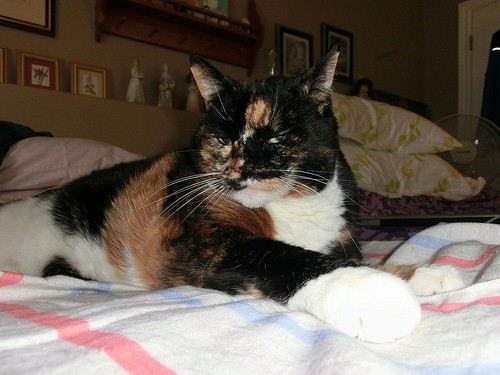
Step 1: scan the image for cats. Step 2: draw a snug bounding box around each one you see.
[0,44,465,336]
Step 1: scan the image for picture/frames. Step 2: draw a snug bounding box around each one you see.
[0,0,56,38]
[16,48,65,92]
[275,22,314,82]
[320,22,354,86]
[71,60,110,99]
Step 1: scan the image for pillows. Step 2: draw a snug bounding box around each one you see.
[331,92,487,202]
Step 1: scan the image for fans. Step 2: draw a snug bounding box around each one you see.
[437,113,500,187]
